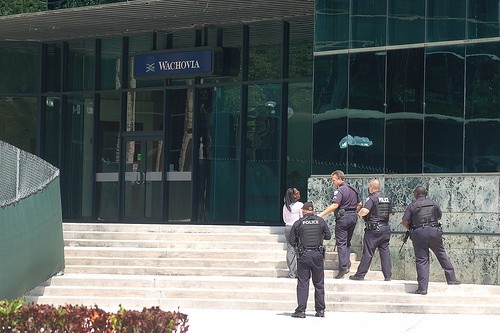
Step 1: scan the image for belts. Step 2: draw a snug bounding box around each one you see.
[306,247,317,251]
[345,208,356,211]
[418,224,438,227]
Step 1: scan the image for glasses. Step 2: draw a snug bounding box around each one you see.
[294,191,299,195]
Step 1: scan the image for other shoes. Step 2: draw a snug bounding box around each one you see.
[385,277,391,280]
[315,313,324,317]
[349,275,364,280]
[448,281,462,284]
[335,269,349,279]
[291,312,305,318]
[415,289,427,294]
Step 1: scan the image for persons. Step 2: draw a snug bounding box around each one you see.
[401,186,461,295]
[349,179,393,281]
[283,188,306,279]
[289,202,331,318]
[318,170,362,278]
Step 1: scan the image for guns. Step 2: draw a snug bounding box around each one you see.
[399,230,410,253]
[333,237,337,252]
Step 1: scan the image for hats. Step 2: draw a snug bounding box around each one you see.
[301,201,314,211]
